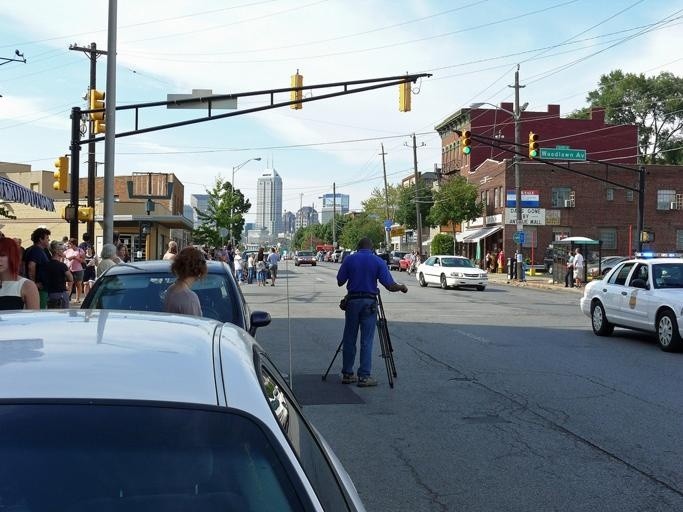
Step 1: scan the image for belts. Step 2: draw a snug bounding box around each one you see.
[347,290,379,300]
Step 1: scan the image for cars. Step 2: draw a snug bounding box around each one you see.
[324,250,333,262]
[399,254,428,272]
[588,257,622,276]
[416,255,488,291]
[295,251,316,266]
[80,261,271,337]
[331,250,342,262]
[0,310,363,512]
[580,252,683,352]
[601,257,635,275]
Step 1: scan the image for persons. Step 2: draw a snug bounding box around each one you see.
[0,238,40,310]
[45,240,73,309]
[564,251,575,288]
[165,246,208,317]
[82,245,98,297]
[407,249,421,275]
[116,243,131,263]
[163,241,177,260]
[97,244,117,278]
[78,233,91,252]
[336,237,408,387]
[63,236,69,246]
[187,241,192,246]
[573,248,584,288]
[253,248,268,288]
[234,251,245,283]
[66,238,85,303]
[22,228,52,309]
[200,243,235,272]
[267,247,280,286]
[247,253,254,284]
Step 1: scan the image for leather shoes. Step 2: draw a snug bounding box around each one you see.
[356,376,377,387]
[341,373,357,384]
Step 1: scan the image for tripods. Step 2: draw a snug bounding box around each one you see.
[321,288,397,389]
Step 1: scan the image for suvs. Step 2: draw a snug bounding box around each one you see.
[544,247,569,274]
[387,250,409,270]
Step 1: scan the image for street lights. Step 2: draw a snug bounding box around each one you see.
[469,102,523,282]
[318,197,336,250]
[230,157,261,246]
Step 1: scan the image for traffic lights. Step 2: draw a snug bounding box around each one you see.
[87,89,105,121]
[92,116,105,135]
[53,157,68,192]
[399,78,412,112]
[529,131,539,160]
[462,130,472,154]
[62,205,94,223]
[291,69,303,109]
